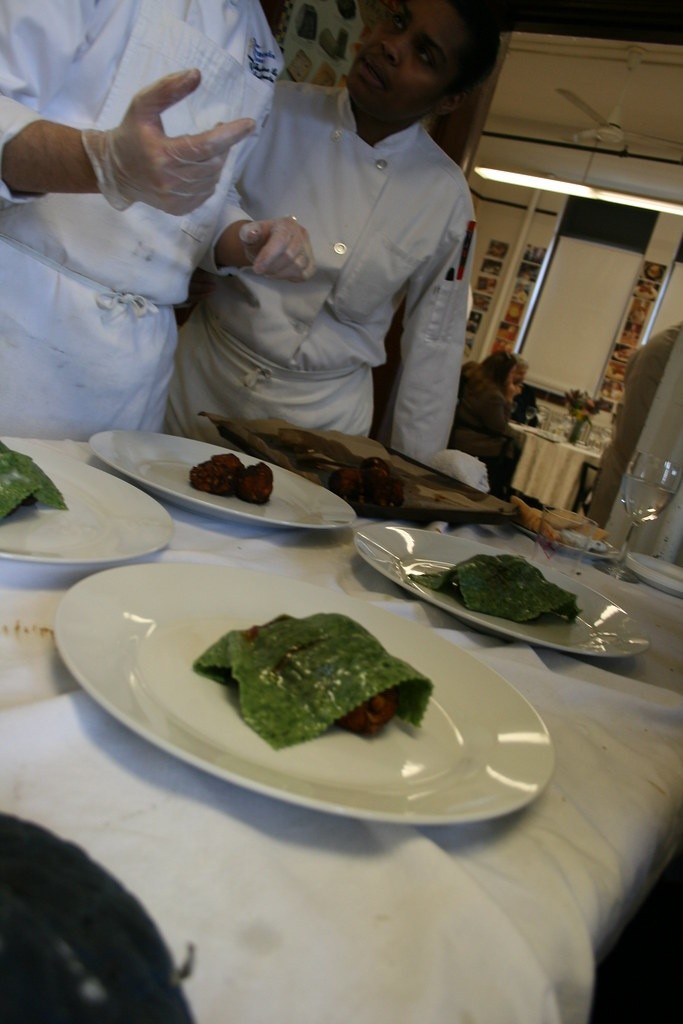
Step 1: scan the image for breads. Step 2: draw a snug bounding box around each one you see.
[511,494,568,543]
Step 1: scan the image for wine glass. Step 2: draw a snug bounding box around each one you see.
[521,406,536,429]
[593,449,683,587]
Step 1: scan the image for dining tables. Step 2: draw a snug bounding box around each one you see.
[506,421,605,515]
[0,437,683,1024]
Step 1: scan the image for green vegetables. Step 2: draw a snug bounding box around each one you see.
[407,552,584,623]
[0,439,68,523]
[193,612,432,747]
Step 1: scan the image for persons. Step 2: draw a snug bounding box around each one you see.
[0,0,317,442]
[162,0,502,466]
[586,323,683,529]
[447,351,539,502]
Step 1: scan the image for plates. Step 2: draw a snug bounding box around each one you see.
[353,526,652,657]
[54,562,554,823]
[88,429,357,530]
[625,553,683,598]
[0,435,173,564]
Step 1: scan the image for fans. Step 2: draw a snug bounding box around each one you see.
[553,43,683,153]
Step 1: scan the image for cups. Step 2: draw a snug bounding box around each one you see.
[529,504,598,576]
